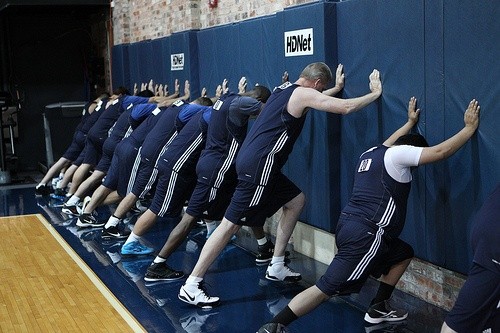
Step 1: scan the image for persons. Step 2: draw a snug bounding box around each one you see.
[177,60,382,309]
[254,96,481,333]
[35,76,247,254]
[439,179,500,333]
[144,69,290,282]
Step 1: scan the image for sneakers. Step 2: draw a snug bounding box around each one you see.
[62,203,86,217]
[82,196,91,213]
[364,317,408,333]
[179,308,222,331]
[120,240,154,255]
[177,284,220,306]
[145,278,186,296]
[265,261,302,282]
[62,197,69,206]
[267,295,290,314]
[256,240,289,263]
[130,200,148,212]
[35,185,52,197]
[101,226,128,240]
[364,296,408,323]
[38,199,124,265]
[256,323,288,333]
[123,215,139,227]
[144,263,184,280]
[75,213,105,228]
[54,189,66,199]
[122,257,154,273]
